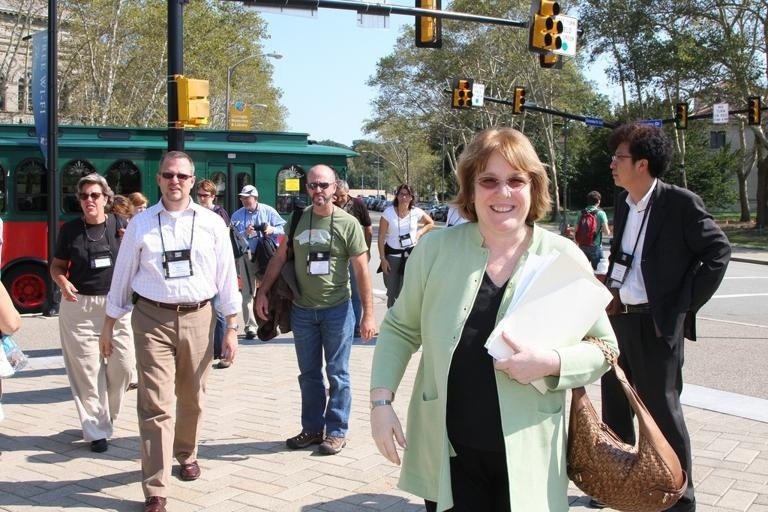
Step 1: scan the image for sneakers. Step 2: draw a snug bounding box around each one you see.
[319,436,347,455]
[287,431,323,449]
[91,438,109,452]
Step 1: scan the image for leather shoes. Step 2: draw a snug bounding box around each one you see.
[145,496,166,512]
[180,461,201,480]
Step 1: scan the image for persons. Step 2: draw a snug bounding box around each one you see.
[193,179,287,368]
[253,166,374,455]
[98,151,243,511]
[50,172,137,453]
[573,190,612,271]
[370,125,622,512]
[588,123,732,512]
[378,184,436,309]
[106,193,148,389]
[446,205,470,226]
[0,213,28,378]
[332,179,372,338]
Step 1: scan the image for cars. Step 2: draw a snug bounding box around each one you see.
[344,189,451,223]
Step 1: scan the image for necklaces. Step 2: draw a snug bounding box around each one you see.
[83,215,108,241]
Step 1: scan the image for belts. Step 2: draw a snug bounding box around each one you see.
[621,304,652,314]
[140,295,208,313]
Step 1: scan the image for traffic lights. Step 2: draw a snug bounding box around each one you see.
[746,96,760,126]
[175,75,209,125]
[529,2,563,52]
[676,103,688,129]
[449,77,474,111]
[513,84,525,115]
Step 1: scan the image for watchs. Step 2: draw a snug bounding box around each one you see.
[227,324,240,331]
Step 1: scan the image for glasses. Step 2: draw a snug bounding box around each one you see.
[612,155,632,161]
[306,182,333,190]
[474,176,533,190]
[79,192,103,200]
[162,172,193,181]
[197,193,211,198]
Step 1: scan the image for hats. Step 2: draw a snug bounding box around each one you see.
[238,185,258,197]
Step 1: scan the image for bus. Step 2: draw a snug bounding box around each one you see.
[0,121,362,314]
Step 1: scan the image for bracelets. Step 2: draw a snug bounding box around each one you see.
[370,399,391,410]
[380,255,385,259]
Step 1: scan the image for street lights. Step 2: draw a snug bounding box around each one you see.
[223,50,284,131]
[360,148,380,189]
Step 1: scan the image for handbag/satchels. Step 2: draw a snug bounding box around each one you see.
[230,225,250,259]
[565,388,688,512]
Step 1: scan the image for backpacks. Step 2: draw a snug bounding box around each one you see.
[575,209,599,246]
